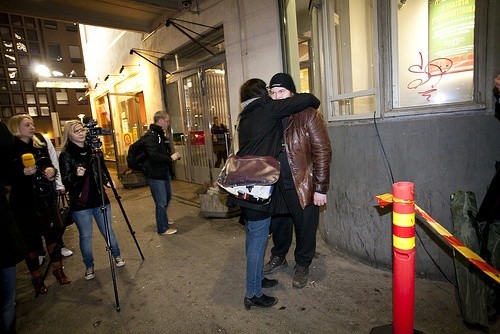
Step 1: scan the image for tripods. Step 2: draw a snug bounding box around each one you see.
[35,147,144,312]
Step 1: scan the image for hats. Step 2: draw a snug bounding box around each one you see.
[269,73,297,94]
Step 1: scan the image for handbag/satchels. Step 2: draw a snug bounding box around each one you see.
[216,153,280,205]
[57,189,74,226]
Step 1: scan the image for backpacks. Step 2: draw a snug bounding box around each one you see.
[126,131,161,172]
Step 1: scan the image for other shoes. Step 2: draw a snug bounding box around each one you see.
[162,228,177,234]
[61,247,73,257]
[84,265,95,280]
[52,268,72,284]
[167,216,174,224]
[114,256,126,267]
[31,276,48,294]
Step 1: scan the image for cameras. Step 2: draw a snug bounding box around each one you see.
[78,120,111,149]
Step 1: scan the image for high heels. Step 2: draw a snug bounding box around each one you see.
[261,278,278,288]
[244,294,278,309]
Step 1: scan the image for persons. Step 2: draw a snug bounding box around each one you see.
[261,73,332,287]
[211,117,231,168]
[141,111,181,235]
[0,114,76,334]
[238,78,321,311]
[59,120,125,280]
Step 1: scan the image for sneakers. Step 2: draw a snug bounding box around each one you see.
[292,263,310,288]
[263,256,289,275]
